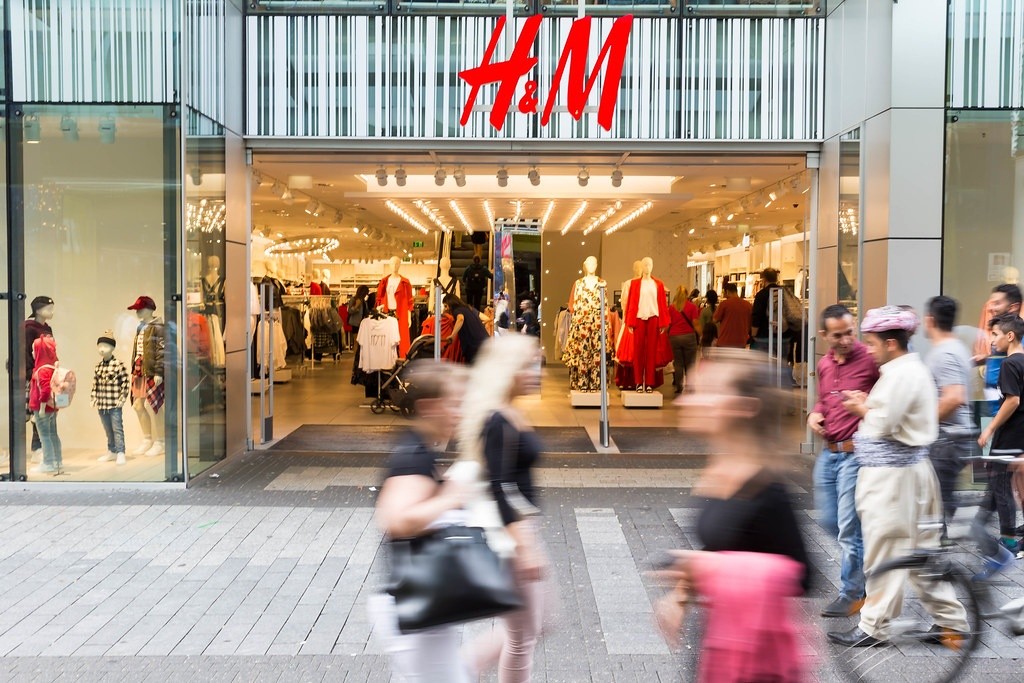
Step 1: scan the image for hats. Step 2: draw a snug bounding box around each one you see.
[95,328,116,347]
[126,296,156,311]
[29,296,54,318]
[861,306,916,334]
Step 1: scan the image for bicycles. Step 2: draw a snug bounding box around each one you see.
[823,449,1023,682]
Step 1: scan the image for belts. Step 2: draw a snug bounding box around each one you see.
[828,441,855,453]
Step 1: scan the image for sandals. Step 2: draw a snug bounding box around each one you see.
[581,385,587,392]
[590,386,596,393]
[646,385,653,393]
[636,384,643,393]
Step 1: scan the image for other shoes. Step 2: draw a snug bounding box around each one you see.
[144,442,164,456]
[33,448,43,456]
[132,439,153,454]
[791,380,800,388]
[116,452,125,464]
[822,525,1023,653]
[30,467,63,477]
[98,451,116,461]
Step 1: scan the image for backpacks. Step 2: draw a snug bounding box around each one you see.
[468,264,487,289]
[35,361,76,409]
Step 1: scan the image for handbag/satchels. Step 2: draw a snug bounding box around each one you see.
[782,286,808,332]
[696,332,700,346]
[377,525,526,635]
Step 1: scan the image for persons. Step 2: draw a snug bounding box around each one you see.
[427,256,461,316]
[442,294,491,365]
[458,330,552,683]
[346,285,369,356]
[260,259,288,371]
[319,270,331,295]
[567,254,672,393]
[462,254,494,312]
[29,334,63,474]
[199,255,226,319]
[310,268,322,297]
[91,325,130,465]
[646,346,820,683]
[667,268,796,394]
[519,300,541,338]
[369,356,481,683]
[470,231,489,261]
[24,296,56,463]
[374,255,415,357]
[126,295,167,457]
[807,284,1024,648]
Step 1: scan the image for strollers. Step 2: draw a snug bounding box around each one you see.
[370,334,451,419]
